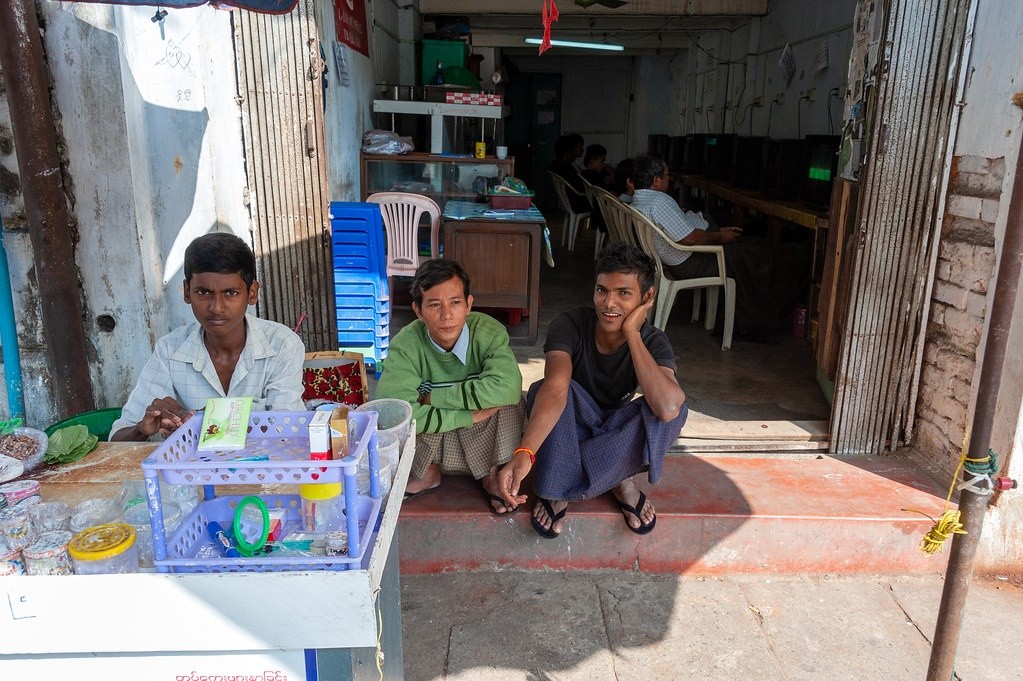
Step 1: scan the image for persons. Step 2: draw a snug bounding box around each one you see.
[499,244,688,539]
[540,133,642,252]
[630,157,811,293]
[107,233,305,441]
[376,259,527,516]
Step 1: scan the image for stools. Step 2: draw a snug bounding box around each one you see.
[329,201,390,382]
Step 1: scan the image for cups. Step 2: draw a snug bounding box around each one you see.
[339,397,413,529]
[21,497,184,575]
[496,146,507,159]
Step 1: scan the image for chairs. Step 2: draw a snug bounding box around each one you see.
[546,167,738,351]
[364,192,442,320]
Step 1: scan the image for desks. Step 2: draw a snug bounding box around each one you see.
[440,199,556,348]
[660,168,832,350]
[0,413,419,681]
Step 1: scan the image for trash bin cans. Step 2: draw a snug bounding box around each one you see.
[792,304,808,338]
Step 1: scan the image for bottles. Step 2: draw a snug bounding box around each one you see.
[298,482,343,531]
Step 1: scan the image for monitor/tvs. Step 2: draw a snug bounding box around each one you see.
[647,133,843,210]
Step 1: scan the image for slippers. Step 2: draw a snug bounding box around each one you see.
[482,487,519,518]
[402,486,441,502]
[532,500,566,540]
[616,489,657,536]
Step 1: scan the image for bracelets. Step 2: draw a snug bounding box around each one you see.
[513,448,535,464]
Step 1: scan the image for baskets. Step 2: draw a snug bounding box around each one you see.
[486,190,535,209]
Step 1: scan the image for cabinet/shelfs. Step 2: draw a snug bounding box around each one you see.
[139,407,389,577]
[421,40,471,104]
[359,150,516,259]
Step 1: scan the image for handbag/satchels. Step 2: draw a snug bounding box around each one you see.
[302,349,369,409]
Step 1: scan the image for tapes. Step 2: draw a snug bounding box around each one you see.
[310,540,327,554]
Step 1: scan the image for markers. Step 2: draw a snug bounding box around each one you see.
[207,519,240,561]
